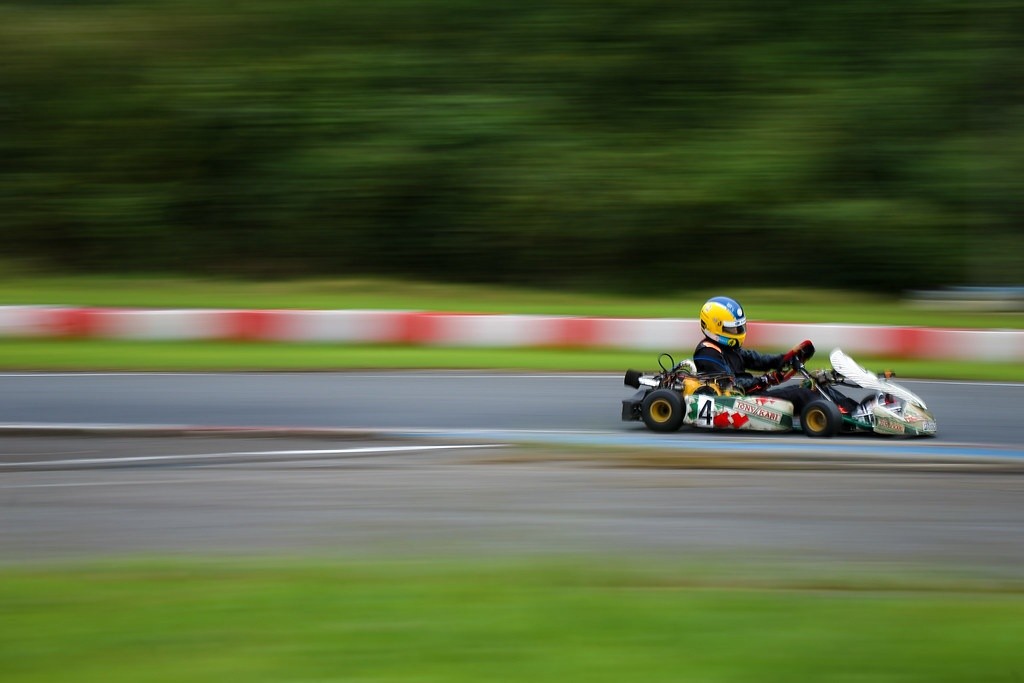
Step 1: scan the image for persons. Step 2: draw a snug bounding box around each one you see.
[694,296,875,415]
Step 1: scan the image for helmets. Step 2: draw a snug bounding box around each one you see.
[700,296,748,348]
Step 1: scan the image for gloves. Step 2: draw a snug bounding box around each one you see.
[783,340,815,365]
[758,368,797,386]
